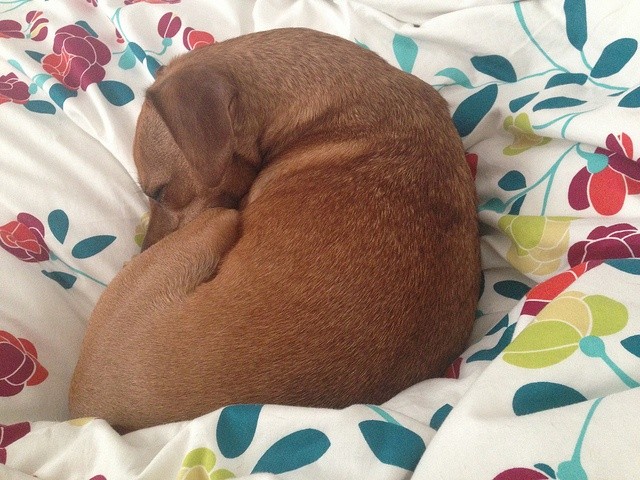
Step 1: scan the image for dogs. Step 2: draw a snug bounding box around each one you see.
[67,27,482,436]
